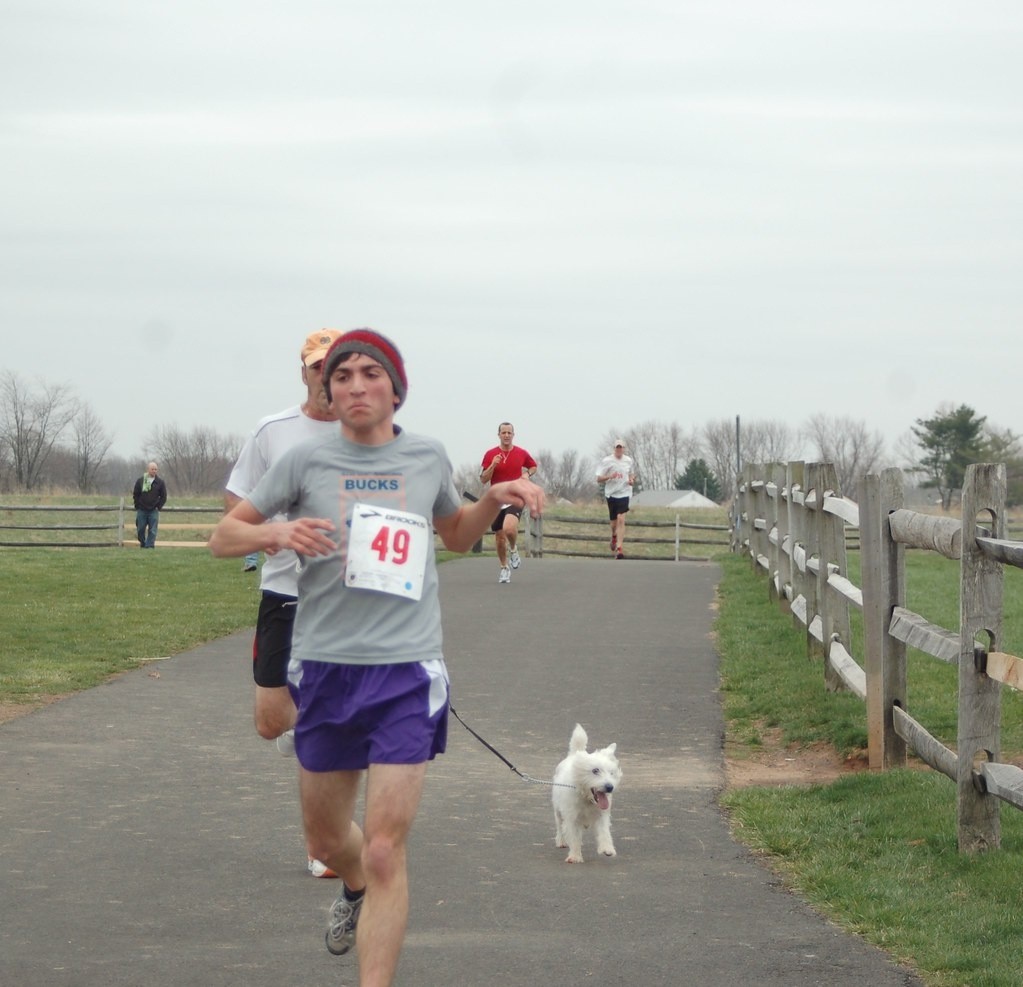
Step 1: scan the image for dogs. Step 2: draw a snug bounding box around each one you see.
[552,721,623,864]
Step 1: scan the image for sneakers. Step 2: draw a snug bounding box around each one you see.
[499,565,511,584]
[610,534,617,551]
[325,880,366,955]
[614,546,624,559]
[508,544,520,569]
[307,854,340,878]
[277,725,296,757]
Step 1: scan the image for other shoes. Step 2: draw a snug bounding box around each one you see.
[241,562,257,573]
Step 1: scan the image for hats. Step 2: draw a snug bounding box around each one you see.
[614,440,625,448]
[321,328,408,412]
[301,330,345,368]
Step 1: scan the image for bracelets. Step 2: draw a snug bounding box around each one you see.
[527,470,532,476]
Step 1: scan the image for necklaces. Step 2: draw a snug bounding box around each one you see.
[501,448,511,464]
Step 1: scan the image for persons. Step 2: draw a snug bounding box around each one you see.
[133,462,167,548]
[480,422,537,582]
[225,329,347,878]
[597,440,635,559]
[209,329,547,987]
